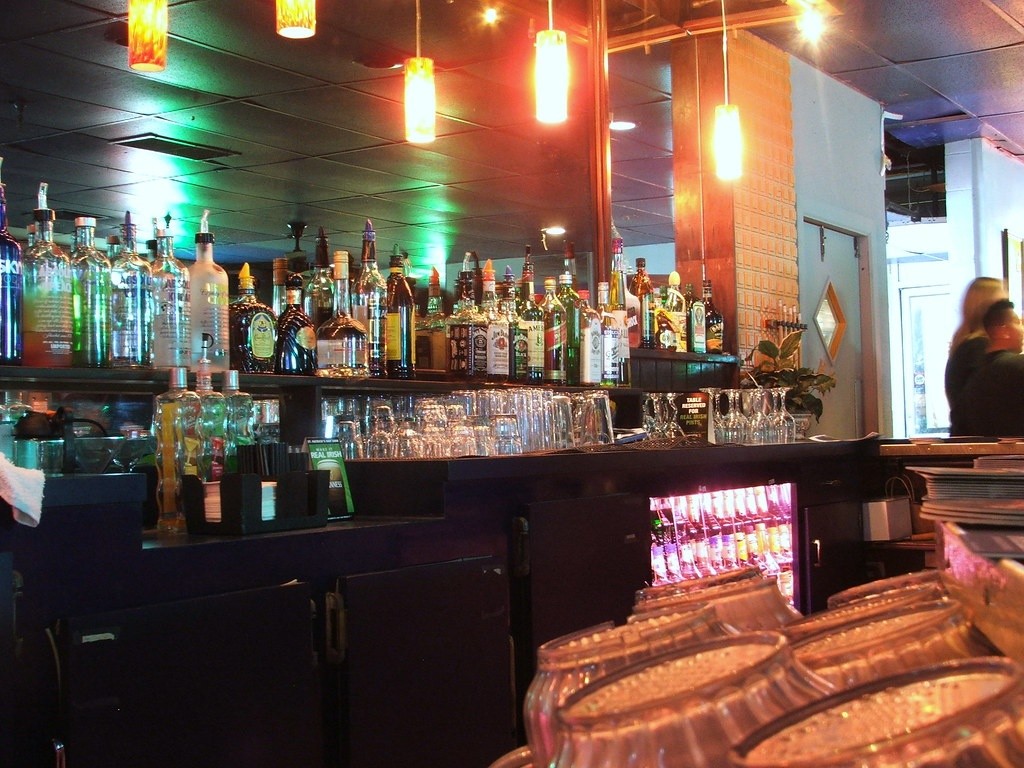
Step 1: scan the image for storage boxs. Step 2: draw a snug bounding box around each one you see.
[863,496,912,540]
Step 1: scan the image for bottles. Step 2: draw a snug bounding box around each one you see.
[230,275,280,374]
[317,250,371,379]
[145,239,157,264]
[419,238,727,388]
[304,228,332,325]
[106,234,120,259]
[189,210,230,373]
[349,218,386,378]
[110,210,155,368]
[150,228,192,370]
[24,209,73,368]
[272,258,288,318]
[25,225,37,252]
[386,243,417,379]
[277,273,318,375]
[69,229,77,260]
[0,185,22,366]
[156,368,258,529]
[651,483,796,607]
[69,217,111,367]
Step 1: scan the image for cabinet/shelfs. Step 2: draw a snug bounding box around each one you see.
[0,456,902,767]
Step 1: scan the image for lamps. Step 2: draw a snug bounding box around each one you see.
[127,0,168,72]
[712,0,745,182]
[276,0,316,39]
[404,0,437,143]
[535,0,570,125]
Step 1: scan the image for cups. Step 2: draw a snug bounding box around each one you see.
[180,471,330,534]
[316,459,348,515]
[40,440,64,478]
[517,569,1024,768]
[337,389,616,456]
[13,441,39,473]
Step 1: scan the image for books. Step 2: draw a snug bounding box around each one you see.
[959,529,1024,558]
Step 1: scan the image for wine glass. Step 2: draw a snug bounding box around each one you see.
[700,387,796,443]
[642,393,689,442]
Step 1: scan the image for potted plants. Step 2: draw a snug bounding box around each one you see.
[740,331,837,440]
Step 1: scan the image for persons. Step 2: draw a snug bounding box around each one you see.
[944,277,1024,438]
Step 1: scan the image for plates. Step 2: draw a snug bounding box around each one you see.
[895,463,1023,528]
[202,480,277,524]
